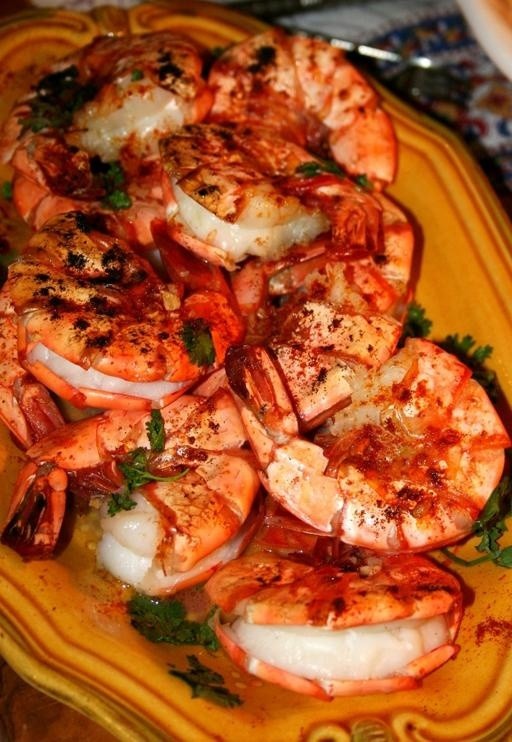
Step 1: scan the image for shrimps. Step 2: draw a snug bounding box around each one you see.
[0,27,512,704]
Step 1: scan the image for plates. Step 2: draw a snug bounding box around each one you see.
[1,1,511,742]
